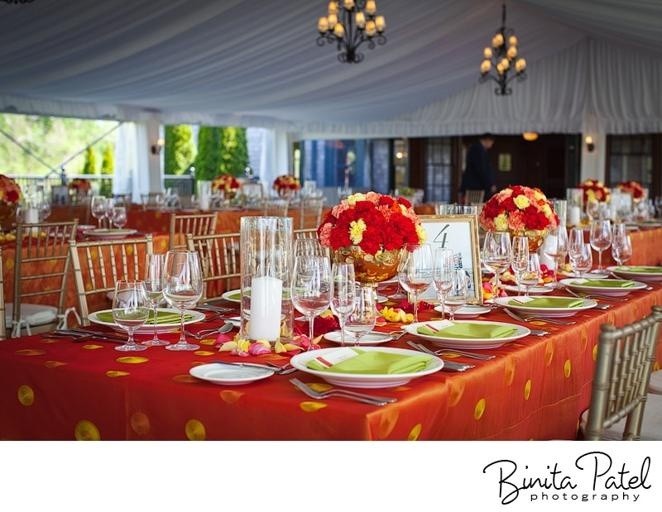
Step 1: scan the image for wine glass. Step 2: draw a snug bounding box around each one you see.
[111,199,662,353]
[91,195,128,229]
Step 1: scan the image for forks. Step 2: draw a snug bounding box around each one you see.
[289,376,398,403]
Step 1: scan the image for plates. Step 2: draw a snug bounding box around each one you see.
[88,262,662,389]
[81,229,137,240]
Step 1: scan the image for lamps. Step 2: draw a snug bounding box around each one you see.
[585,136,595,153]
[315,1,387,65]
[479,1,528,95]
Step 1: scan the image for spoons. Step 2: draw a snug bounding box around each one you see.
[38,332,94,343]
